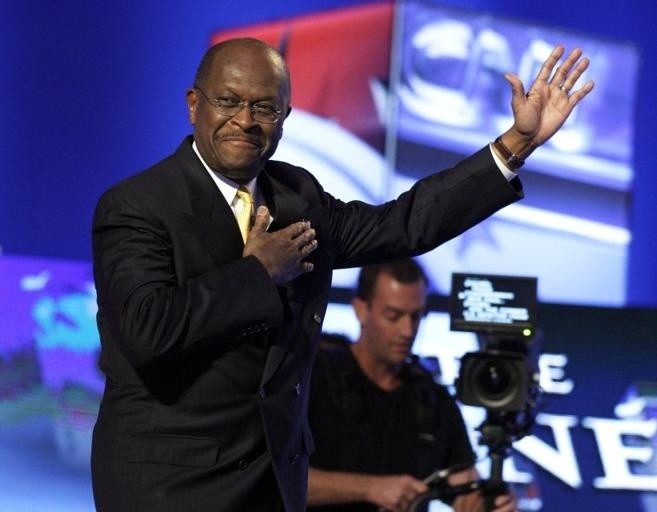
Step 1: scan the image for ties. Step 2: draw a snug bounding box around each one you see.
[236,184,255,247]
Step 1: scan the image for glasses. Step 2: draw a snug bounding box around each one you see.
[194,84,288,125]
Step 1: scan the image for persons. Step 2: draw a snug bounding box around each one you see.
[302,255,519,511]
[87,37,595,511]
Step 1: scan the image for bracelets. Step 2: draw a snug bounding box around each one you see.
[491,136,525,169]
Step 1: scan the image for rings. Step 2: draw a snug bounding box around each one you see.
[559,85,570,93]
